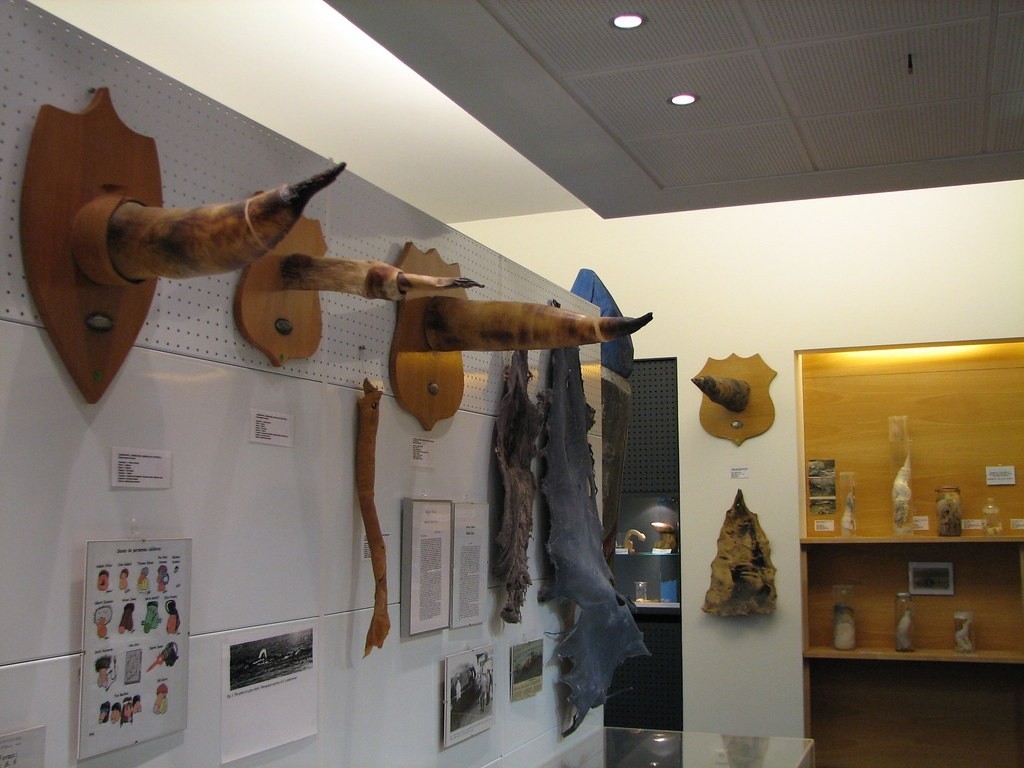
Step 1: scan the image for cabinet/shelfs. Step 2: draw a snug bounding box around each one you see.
[613,551,681,614]
[799,338,1024,768]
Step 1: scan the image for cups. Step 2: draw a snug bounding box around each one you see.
[634,581,647,602]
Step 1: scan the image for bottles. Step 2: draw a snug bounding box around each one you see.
[983,499,1002,535]
